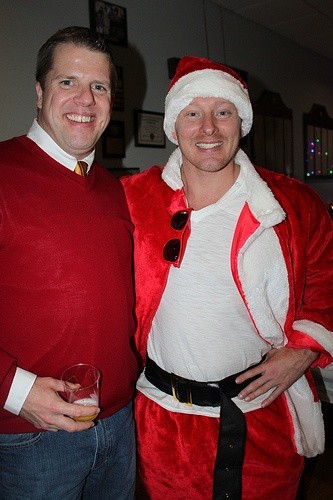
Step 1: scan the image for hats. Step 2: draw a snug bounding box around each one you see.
[163,56,254,145]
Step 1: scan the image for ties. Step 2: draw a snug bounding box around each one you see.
[75,161,88,176]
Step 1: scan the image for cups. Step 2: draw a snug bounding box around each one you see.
[61,363,101,422]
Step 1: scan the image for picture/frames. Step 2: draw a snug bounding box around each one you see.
[112,65,125,112]
[88,0,129,48]
[101,120,125,158]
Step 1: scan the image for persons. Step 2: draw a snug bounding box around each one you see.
[119,56,333,500]
[0,27,140,500]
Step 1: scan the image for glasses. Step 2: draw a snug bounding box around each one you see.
[161,207,193,268]
